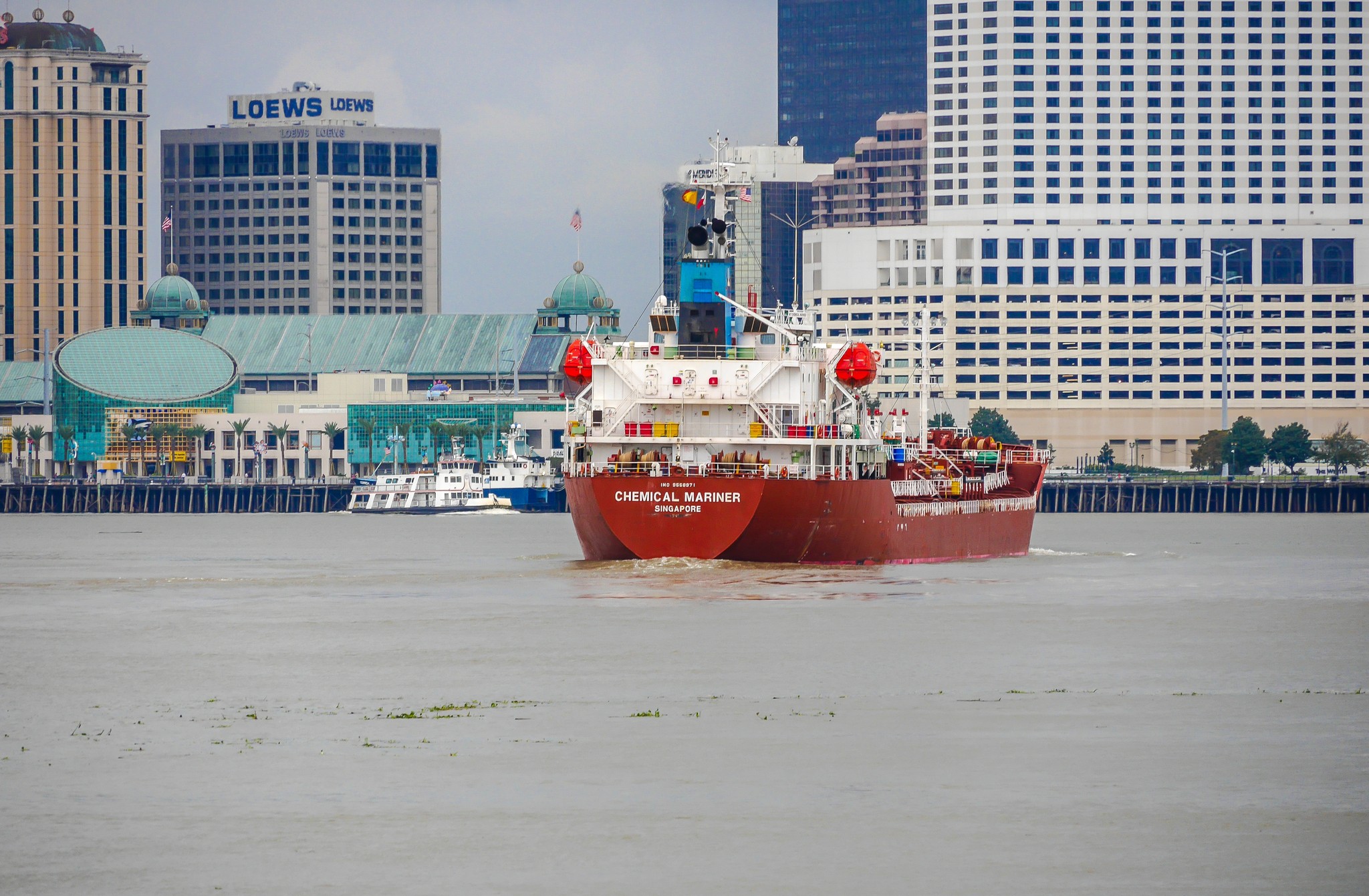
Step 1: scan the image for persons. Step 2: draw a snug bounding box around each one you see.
[1108,469,1130,483]
[54,473,57,479]
[167,473,169,476]
[1292,470,1299,482]
[351,472,359,479]
[86,472,97,483]
[322,474,326,484]
[1060,472,1064,483]
[181,471,186,483]
[245,472,249,483]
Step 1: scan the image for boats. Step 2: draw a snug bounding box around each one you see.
[345,424,568,515]
[560,130,1053,567]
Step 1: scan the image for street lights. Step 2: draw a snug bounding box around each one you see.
[209,442,216,483]
[302,443,309,479]
[1231,442,1238,480]
[1141,454,1144,468]
[91,452,97,479]
[347,449,354,478]
[1129,442,1135,467]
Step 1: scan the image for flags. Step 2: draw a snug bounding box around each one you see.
[569,209,582,232]
[682,188,706,210]
[385,447,391,456]
[162,210,172,234]
[739,188,751,202]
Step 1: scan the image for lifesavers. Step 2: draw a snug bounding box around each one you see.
[580,463,585,474]
[871,351,881,362]
[780,466,788,478]
[523,464,527,468]
[847,379,857,388]
[817,425,823,438]
[576,376,585,386]
[835,466,840,478]
